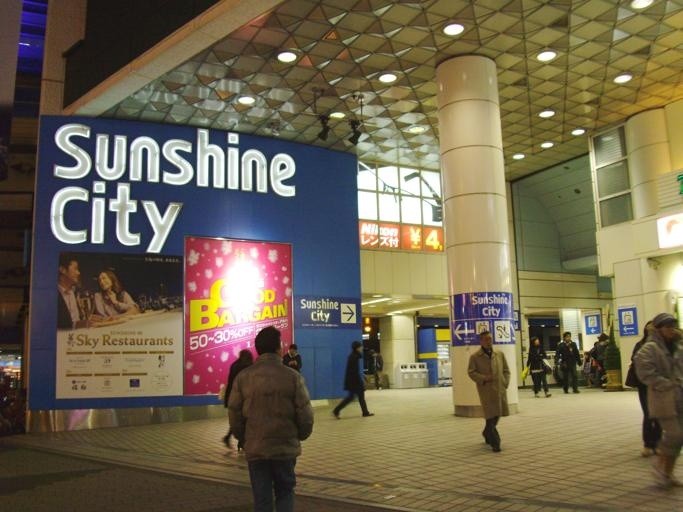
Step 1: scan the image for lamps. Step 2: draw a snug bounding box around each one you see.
[348,94,364,146]
[311,87,331,142]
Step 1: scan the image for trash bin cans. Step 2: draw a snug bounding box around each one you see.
[395,362,429,388]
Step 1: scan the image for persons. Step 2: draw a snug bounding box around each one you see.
[632,312,683,488]
[221,348,253,453]
[526,335,552,398]
[332,340,374,420]
[226,324,313,510]
[281,343,302,371]
[554,331,583,393]
[581,333,620,388]
[56,251,103,330]
[467,331,510,451]
[89,266,136,318]
[368,346,383,390]
[629,322,662,455]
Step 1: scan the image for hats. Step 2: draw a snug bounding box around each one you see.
[652,313,677,328]
